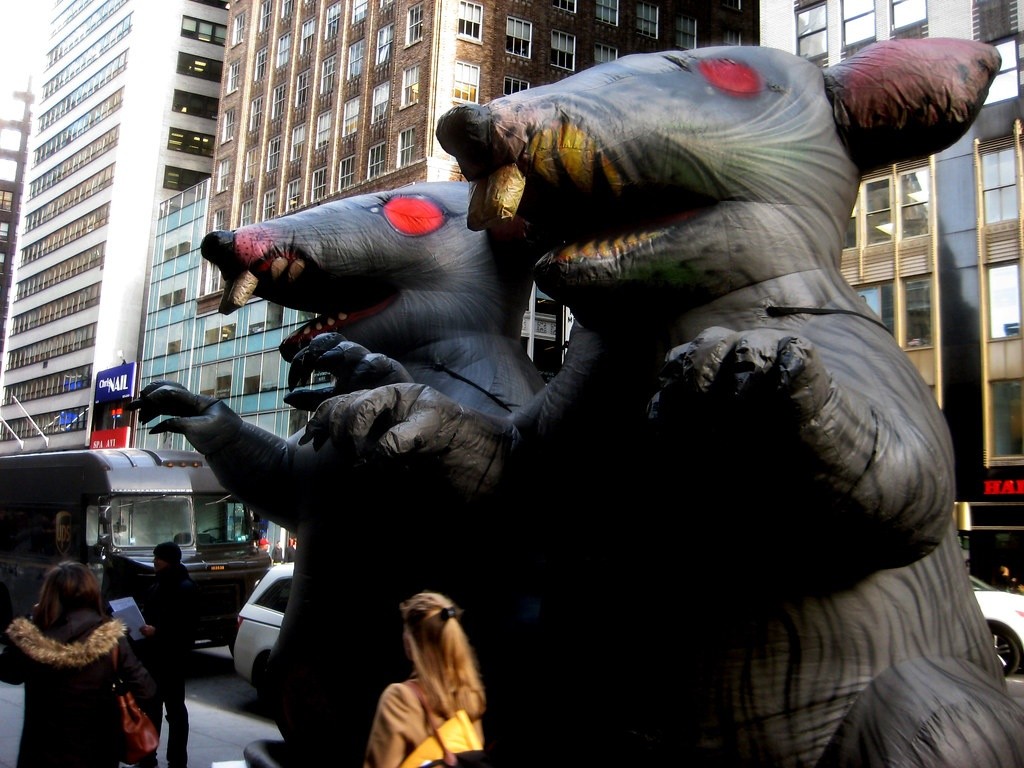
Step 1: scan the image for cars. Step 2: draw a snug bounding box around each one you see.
[967,573,1024,680]
[233,561,295,708]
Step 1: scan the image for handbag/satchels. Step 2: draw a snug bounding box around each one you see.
[398,681,485,768]
[112,644,159,765]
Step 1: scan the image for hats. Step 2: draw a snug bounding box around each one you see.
[154,542,181,565]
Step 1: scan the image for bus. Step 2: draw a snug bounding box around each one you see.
[0,447,273,665]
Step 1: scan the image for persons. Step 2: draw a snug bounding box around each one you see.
[363,591,486,768]
[0,562,158,768]
[999,566,1018,591]
[256,536,298,565]
[123,541,194,768]
